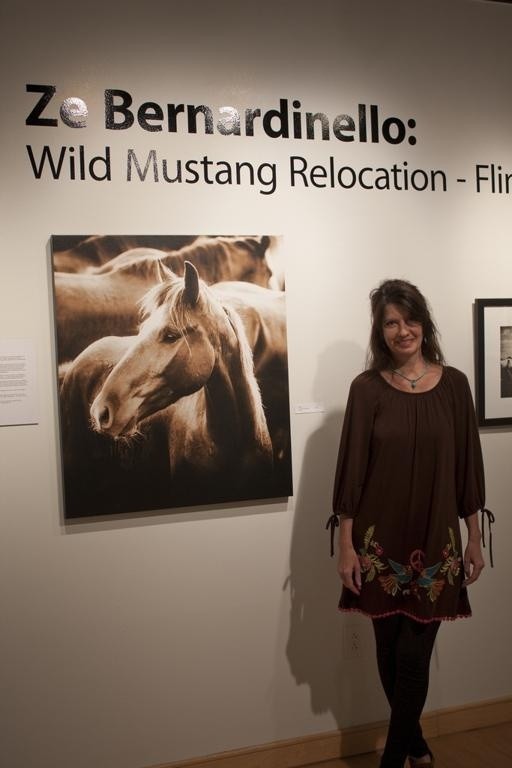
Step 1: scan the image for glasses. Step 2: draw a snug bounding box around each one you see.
[382,318,423,328]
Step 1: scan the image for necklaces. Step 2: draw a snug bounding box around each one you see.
[393,367,427,390]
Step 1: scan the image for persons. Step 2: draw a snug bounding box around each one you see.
[327,278,496,768]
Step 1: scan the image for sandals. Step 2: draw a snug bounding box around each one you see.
[409,752,435,768]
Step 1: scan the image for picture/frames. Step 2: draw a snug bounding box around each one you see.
[474,298,512,425]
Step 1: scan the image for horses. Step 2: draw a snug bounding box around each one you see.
[54,234,288,468]
